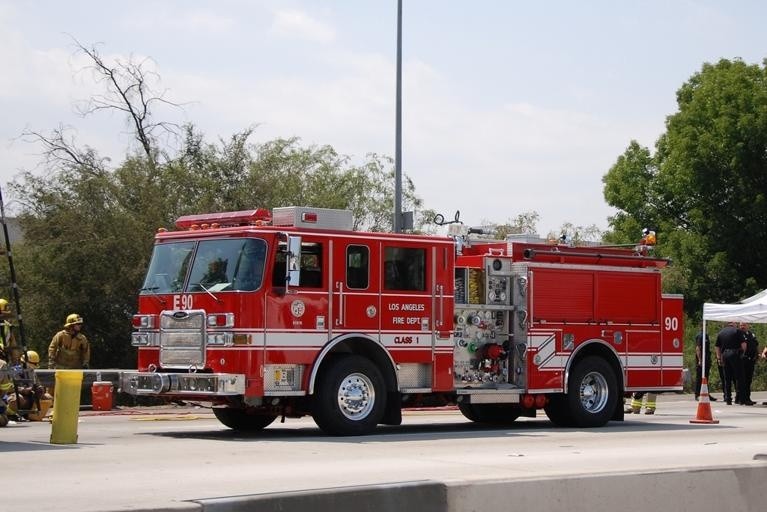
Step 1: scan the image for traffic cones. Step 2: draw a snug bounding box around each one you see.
[690,377,719,424]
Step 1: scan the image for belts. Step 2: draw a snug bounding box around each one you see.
[725,348,736,351]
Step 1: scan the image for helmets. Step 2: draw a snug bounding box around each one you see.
[0,298,13,314]
[20,350,41,369]
[64,313,84,328]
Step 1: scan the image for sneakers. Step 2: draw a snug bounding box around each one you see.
[762,401,767,406]
[645,410,655,414]
[696,395,700,401]
[624,407,641,414]
[710,394,718,401]
[724,397,757,406]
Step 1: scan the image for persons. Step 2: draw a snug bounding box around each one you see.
[0,298,90,428]
[694,320,766,406]
[630,391,656,415]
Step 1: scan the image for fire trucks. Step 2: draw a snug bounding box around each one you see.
[125,205,684,437]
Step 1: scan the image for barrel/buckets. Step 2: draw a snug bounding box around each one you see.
[90,380,114,411]
